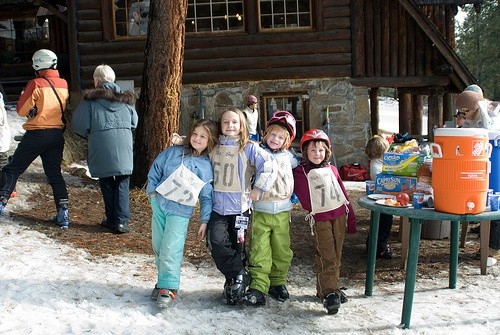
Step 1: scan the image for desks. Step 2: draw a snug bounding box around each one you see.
[357,194,500,330]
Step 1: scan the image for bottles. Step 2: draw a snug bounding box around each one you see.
[129,19,136,35]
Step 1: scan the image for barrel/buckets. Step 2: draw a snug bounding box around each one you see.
[488,129,500,207]
[430,127,492,215]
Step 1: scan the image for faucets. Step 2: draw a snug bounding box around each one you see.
[325,116,331,131]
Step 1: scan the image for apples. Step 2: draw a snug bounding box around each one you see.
[396,193,413,205]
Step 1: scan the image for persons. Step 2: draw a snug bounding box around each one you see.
[246,111,301,306]
[146,119,220,307]
[241,95,259,141]
[71,65,138,233]
[128,6,149,36]
[170,108,278,306]
[0,49,70,229]
[366,134,394,259]
[454,85,500,260]
[0,92,17,197]
[292,128,357,314]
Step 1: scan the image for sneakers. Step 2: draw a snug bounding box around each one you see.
[268,285,290,302]
[316,291,348,303]
[223,270,249,304]
[151,284,159,300]
[323,293,341,315]
[245,289,266,306]
[157,290,174,307]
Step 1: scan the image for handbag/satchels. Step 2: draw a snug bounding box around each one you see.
[339,163,368,182]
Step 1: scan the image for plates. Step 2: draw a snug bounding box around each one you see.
[375,199,412,207]
[367,194,397,200]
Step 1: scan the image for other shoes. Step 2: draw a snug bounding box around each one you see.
[376,244,393,257]
[53,213,69,228]
[102,219,129,233]
[470,224,480,232]
[0,201,6,212]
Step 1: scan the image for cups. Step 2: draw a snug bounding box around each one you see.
[487,188,500,211]
[366,182,375,195]
[413,193,424,209]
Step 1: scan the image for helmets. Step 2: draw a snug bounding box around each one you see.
[300,129,331,153]
[268,111,296,138]
[32,49,57,70]
[248,95,257,103]
[456,91,478,109]
[464,85,482,95]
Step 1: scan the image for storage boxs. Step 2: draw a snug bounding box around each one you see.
[382,153,425,176]
[376,174,433,195]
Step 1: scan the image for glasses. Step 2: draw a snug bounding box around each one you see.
[456,110,469,118]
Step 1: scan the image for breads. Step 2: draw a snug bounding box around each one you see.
[385,199,394,204]
